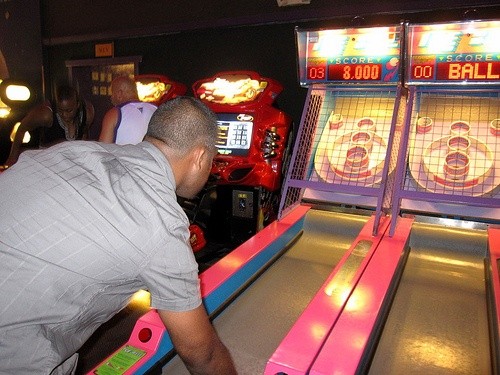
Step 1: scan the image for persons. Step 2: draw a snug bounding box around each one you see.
[0,97,235,375]
[3,85,95,168]
[99,78,160,145]
